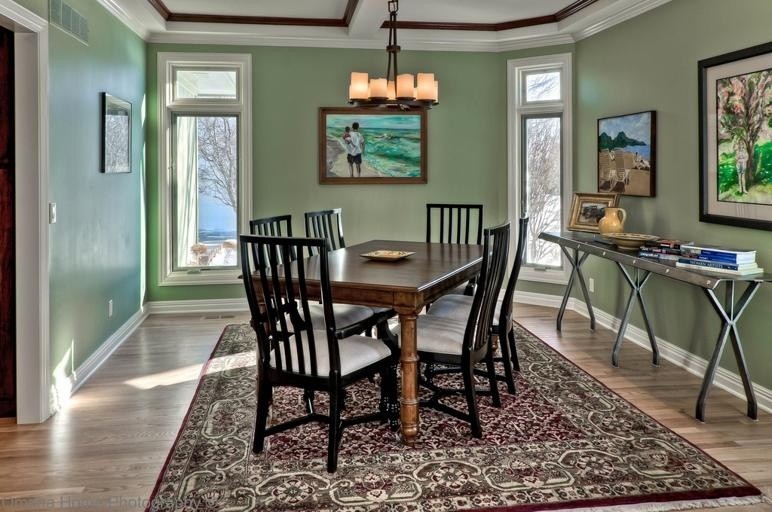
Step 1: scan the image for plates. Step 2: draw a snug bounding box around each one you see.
[360,248,417,263]
[600,231,660,251]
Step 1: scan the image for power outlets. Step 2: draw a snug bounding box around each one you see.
[589,278,595,293]
[108,299,113,318]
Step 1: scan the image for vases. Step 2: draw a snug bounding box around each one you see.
[599,208,627,234]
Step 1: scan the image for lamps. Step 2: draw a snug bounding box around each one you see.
[348,0,439,112]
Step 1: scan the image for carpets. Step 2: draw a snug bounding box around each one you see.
[145,319,772,512]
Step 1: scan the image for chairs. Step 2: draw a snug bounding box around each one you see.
[239,234,399,473]
[426,217,530,396]
[249,214,375,413]
[390,221,510,438]
[304,208,398,384]
[426,204,484,315]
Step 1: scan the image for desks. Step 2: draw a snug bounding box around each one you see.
[538,232,772,422]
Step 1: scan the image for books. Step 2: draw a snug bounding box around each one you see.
[639,239,694,261]
[674,244,765,277]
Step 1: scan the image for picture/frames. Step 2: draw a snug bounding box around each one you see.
[319,107,428,185]
[101,92,132,174]
[566,192,621,234]
[597,110,657,198]
[697,41,772,231]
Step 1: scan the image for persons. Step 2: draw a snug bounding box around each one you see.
[736,141,748,193]
[343,127,352,144]
[344,122,364,177]
